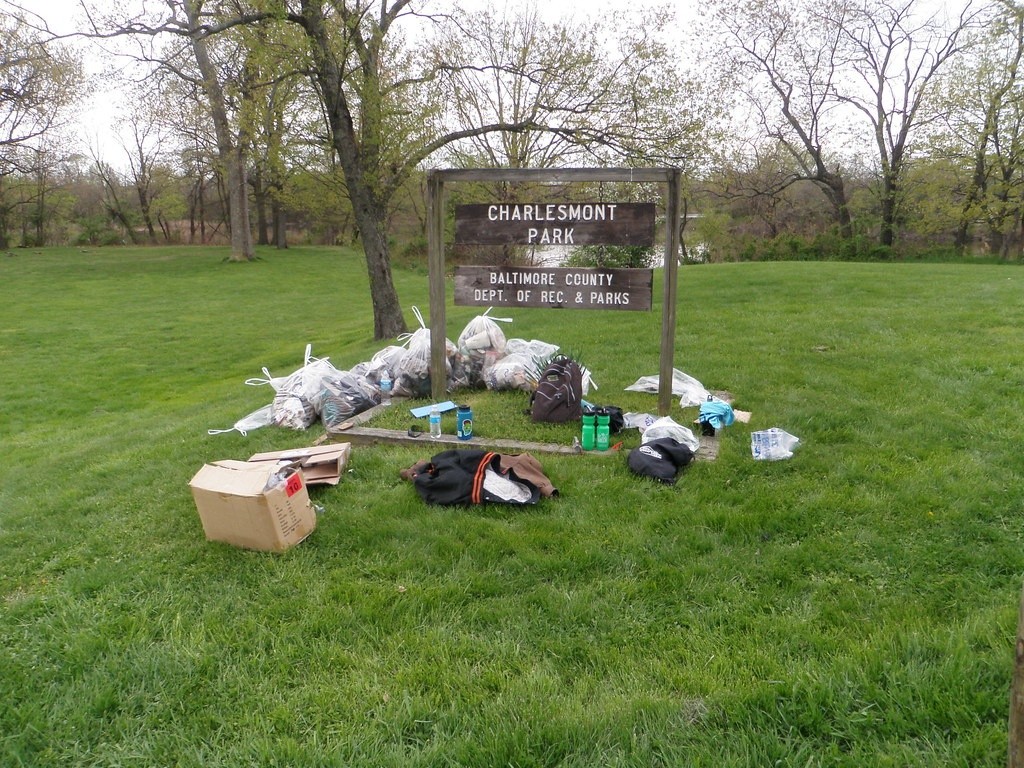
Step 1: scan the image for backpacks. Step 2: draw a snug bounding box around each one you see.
[530,353,583,422]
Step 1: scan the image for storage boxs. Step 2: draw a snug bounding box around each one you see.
[188,461,316,558]
[249,441,350,486]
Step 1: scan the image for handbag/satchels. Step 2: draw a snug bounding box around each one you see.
[627,437,694,482]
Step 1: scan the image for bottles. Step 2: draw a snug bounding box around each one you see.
[456,405,472,440]
[380,370,392,406]
[595,406,609,451]
[582,404,595,450]
[430,405,442,440]
